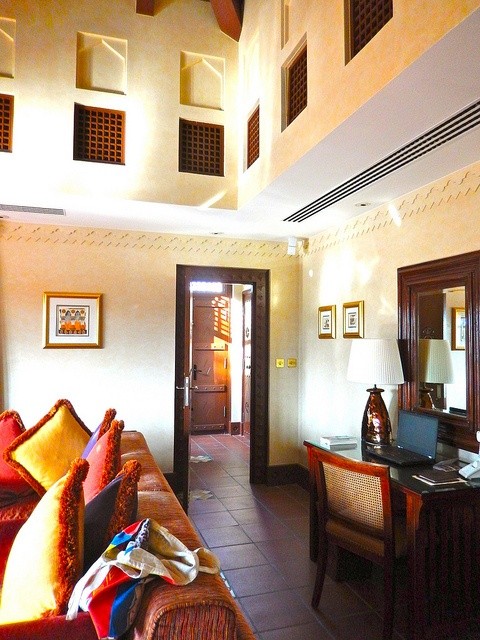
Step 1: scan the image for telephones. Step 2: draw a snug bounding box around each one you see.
[433,456,472,473]
[460,458,480,479]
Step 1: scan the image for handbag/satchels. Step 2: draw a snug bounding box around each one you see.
[65,517,223,640]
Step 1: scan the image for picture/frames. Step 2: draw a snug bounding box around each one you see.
[340,301,365,337]
[316,305,337,341]
[452,306,468,351]
[40,291,104,348]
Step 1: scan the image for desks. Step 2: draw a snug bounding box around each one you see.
[302,437,480,636]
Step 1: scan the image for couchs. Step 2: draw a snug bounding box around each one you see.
[0,430,253,640]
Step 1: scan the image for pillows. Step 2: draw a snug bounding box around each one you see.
[0,410,35,498]
[80,407,116,456]
[3,397,94,496]
[83,457,143,573]
[81,420,125,502]
[0,457,90,617]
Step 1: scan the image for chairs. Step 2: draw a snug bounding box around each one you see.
[307,444,407,638]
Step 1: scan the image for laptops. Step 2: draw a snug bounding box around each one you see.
[366,410,439,467]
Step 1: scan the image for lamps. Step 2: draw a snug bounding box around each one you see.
[418,338,455,409]
